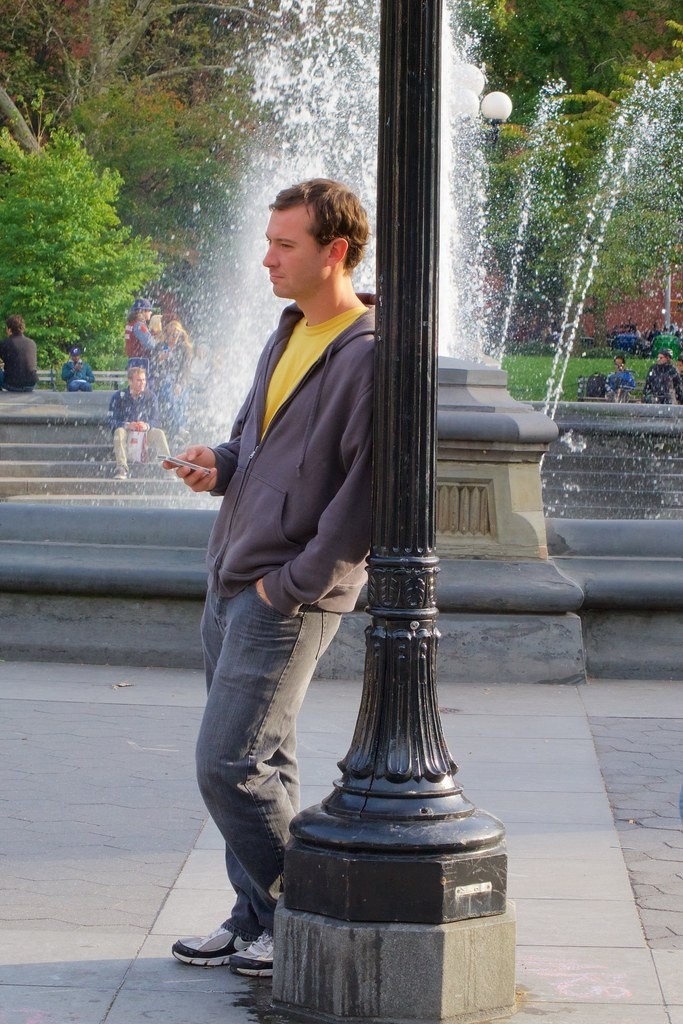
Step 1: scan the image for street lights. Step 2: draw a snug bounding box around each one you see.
[439,64,513,357]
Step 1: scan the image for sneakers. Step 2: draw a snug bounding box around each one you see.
[172,919,258,966]
[229,931,273,976]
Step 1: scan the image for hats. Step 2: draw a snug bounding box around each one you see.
[131,299,152,309]
[659,349,673,359]
[71,347,81,356]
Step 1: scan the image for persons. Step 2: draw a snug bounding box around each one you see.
[166,179,375,979]
[604,355,636,403]
[125,296,211,450]
[0,313,39,392]
[674,354,683,405]
[61,347,95,392]
[605,321,683,362]
[105,365,179,480]
[640,348,683,405]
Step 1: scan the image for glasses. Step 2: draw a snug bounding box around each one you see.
[657,357,662,359]
[167,333,178,338]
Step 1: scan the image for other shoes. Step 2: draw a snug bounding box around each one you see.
[114,471,127,480]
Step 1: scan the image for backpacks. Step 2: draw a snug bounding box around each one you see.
[586,372,607,397]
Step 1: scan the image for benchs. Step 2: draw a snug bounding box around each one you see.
[87,371,129,391]
[2,365,58,391]
[577,375,646,402]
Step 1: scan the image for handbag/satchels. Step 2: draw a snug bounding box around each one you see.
[127,425,149,463]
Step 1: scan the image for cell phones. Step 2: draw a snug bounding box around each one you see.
[157,455,210,475]
